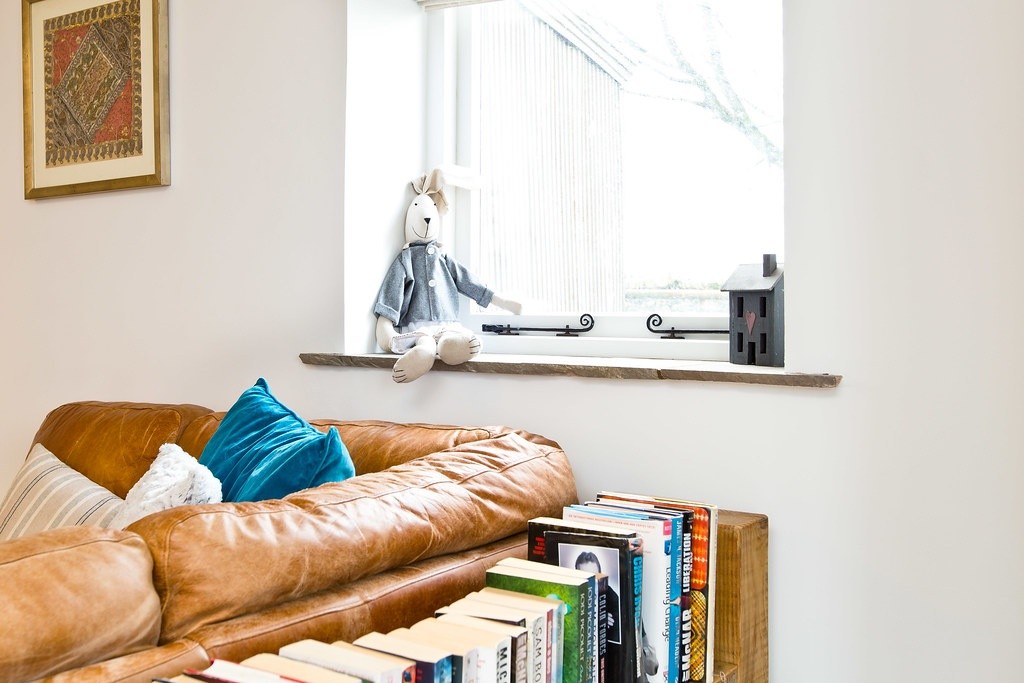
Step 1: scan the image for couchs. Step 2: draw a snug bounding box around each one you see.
[0,400,581,683]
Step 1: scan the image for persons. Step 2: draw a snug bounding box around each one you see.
[576,552,618,641]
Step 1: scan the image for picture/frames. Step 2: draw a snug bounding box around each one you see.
[22,0,171,199]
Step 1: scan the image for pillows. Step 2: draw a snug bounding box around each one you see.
[0,442,124,542]
[197,377,356,505]
[110,443,223,531]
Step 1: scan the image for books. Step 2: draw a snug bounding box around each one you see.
[155,491,719,683]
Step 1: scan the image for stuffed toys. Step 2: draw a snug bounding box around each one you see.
[374,169,523,383]
